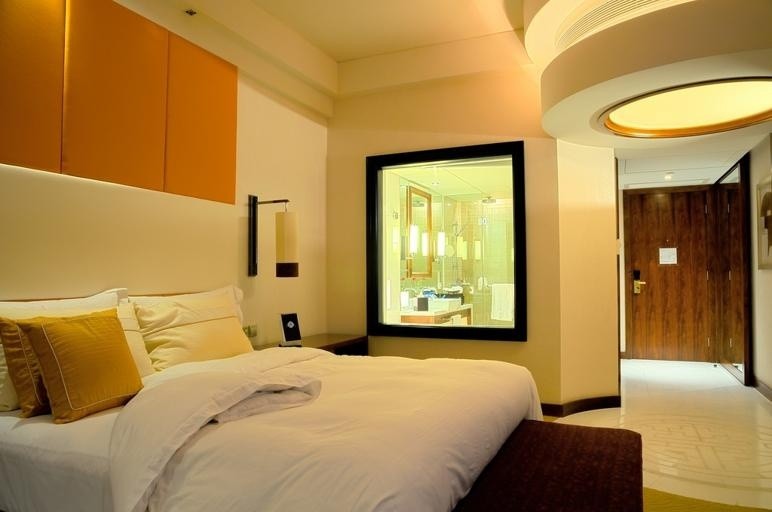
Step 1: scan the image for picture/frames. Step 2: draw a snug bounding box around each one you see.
[755,175,772,270]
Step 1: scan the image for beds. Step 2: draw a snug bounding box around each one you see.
[1,291,546,512]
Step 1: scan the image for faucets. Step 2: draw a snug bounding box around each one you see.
[404,287,424,297]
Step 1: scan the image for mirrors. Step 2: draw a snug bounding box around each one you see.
[406,186,433,278]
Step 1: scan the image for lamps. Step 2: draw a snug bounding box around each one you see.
[246,193,299,278]
[405,224,419,260]
[436,224,446,264]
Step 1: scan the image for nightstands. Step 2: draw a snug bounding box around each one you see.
[255,332,370,359]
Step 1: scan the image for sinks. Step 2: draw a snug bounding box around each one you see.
[412,297,462,312]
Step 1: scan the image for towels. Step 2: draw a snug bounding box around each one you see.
[491,283,515,322]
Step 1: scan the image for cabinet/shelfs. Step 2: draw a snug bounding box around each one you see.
[401,308,473,325]
[0,0,238,206]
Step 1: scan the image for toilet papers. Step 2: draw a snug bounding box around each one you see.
[400,291,409,308]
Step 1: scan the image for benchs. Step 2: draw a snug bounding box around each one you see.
[451,418,644,511]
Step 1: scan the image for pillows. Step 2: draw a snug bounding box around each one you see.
[1,287,129,412]
[119,301,157,376]
[129,282,254,373]
[1,317,55,419]
[17,307,144,425]
